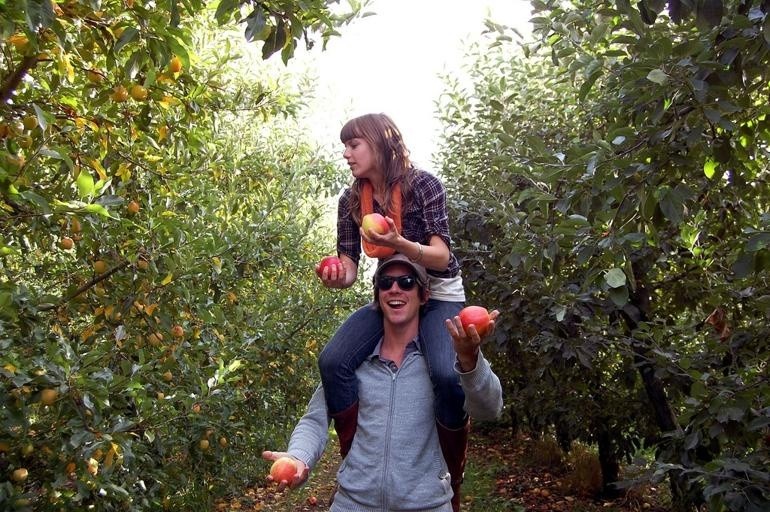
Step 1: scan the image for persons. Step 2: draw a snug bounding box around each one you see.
[260,252,503,512]
[314,110,470,512]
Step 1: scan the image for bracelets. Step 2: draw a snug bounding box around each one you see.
[407,240,423,263]
[325,284,346,293]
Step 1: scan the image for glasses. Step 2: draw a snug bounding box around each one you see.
[376,274,416,290]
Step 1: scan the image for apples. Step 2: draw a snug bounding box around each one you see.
[0,0,551,512]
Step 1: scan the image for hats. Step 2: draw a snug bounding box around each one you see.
[373,253,430,289]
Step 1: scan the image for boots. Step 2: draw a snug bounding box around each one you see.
[435,414,472,512]
[328,399,358,458]
